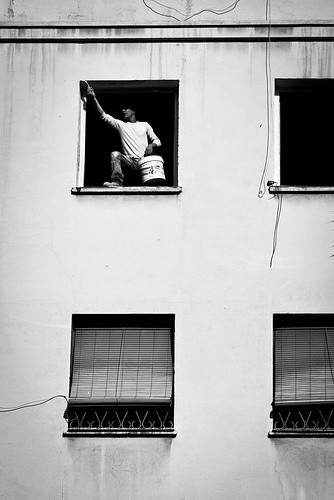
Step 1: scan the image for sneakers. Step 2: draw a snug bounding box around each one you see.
[103,181,123,187]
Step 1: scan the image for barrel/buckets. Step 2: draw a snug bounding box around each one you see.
[138,156,166,185]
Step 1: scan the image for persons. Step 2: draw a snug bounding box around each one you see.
[86,85,162,188]
